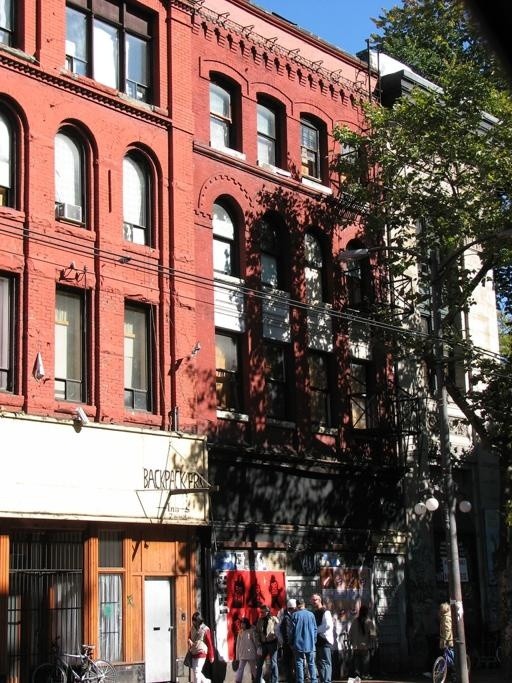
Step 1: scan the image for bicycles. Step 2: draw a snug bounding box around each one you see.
[30,633,116,682]
[494,637,511,665]
[432,636,472,683]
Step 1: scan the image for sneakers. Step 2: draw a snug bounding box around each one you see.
[362,673,373,679]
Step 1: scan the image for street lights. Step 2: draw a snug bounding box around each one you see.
[331,228,512,682]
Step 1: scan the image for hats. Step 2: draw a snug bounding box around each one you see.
[288,598,297,608]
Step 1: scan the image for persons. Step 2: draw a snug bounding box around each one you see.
[438,592,465,683]
[246,575,266,625]
[268,575,283,614]
[232,574,246,618]
[348,604,375,679]
[234,593,362,683]
[188,612,215,683]
[231,613,240,660]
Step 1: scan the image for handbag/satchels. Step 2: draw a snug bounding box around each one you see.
[184,649,192,667]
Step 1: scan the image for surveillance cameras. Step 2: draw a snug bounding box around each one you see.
[75,407,89,425]
